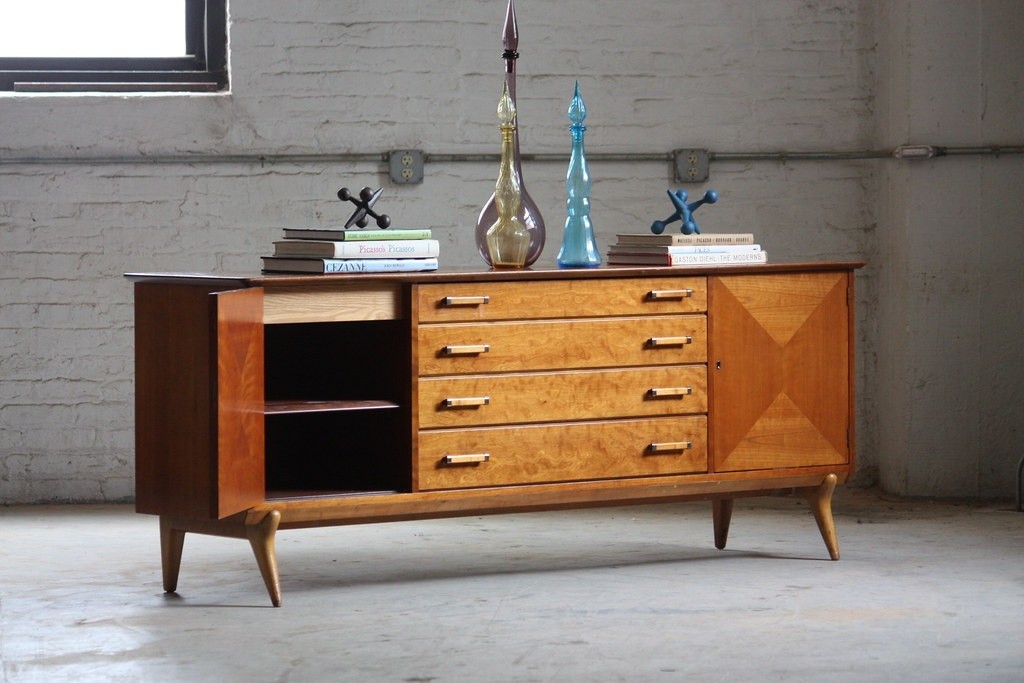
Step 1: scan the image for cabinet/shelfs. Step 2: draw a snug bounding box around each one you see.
[121,258,868,606]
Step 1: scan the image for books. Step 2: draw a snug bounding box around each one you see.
[606,232,768,266]
[260,226,441,274]
